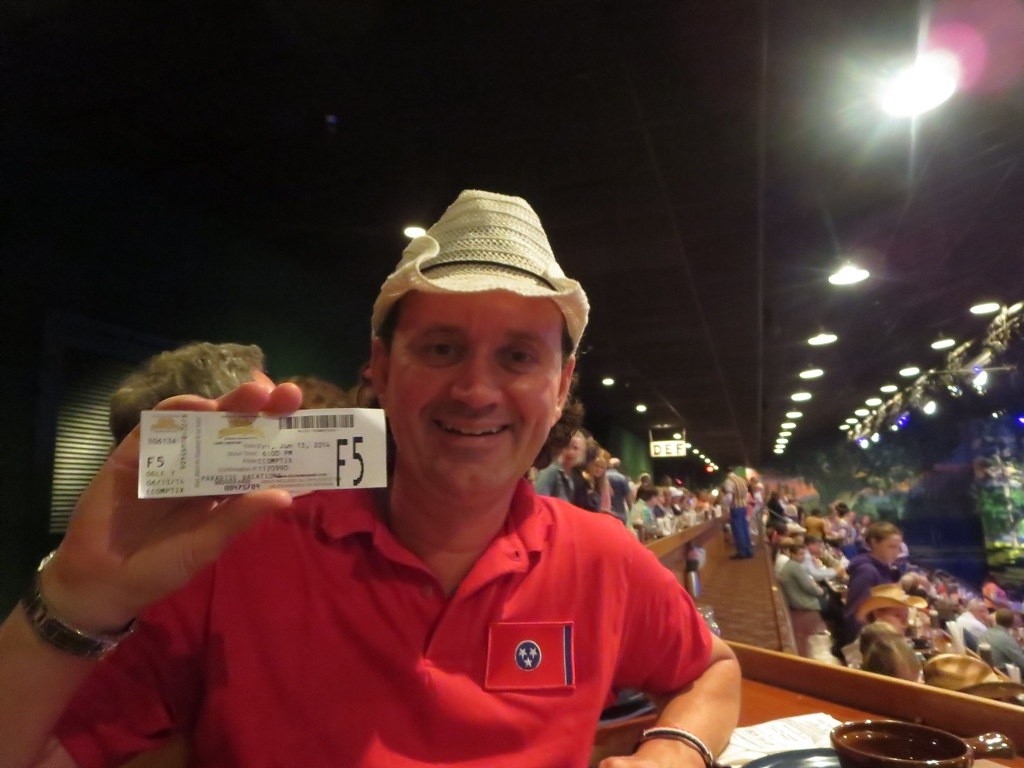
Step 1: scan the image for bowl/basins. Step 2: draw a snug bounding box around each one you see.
[830,720,1015,768]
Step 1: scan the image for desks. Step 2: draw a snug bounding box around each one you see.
[593,636,1024,768]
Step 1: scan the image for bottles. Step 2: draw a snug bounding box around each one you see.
[687,561,700,597]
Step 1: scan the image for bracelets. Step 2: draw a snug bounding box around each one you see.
[20,550,136,659]
[630,725,714,768]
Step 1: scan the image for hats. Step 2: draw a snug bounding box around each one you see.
[371,188,590,355]
[787,522,806,535]
[669,486,684,498]
[856,583,928,626]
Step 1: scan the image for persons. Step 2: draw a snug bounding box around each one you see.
[956,596,989,638]
[854,578,928,637]
[777,541,839,618]
[840,524,923,642]
[801,509,832,544]
[981,570,1012,614]
[0,187,742,768]
[627,487,660,537]
[633,472,650,498]
[767,491,787,519]
[108,341,1024,680]
[605,457,632,521]
[720,465,755,560]
[534,427,585,503]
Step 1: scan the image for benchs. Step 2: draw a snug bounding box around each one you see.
[758,517,841,665]
